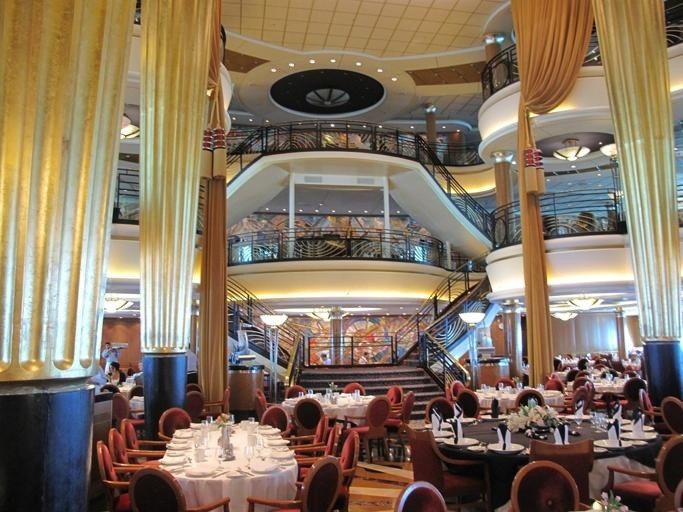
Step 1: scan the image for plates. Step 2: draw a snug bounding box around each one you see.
[161,421,295,478]
[424,413,658,454]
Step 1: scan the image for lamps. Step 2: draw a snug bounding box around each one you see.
[553,139,590,161]
[459,313,484,388]
[567,299,603,310]
[260,315,287,403]
[552,312,576,320]
[120,125,140,139]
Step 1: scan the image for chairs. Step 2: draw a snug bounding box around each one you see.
[95,348,683,512]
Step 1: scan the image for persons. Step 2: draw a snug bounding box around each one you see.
[521,356,530,386]
[358,352,368,364]
[186,342,198,384]
[132,362,143,384]
[320,352,331,365]
[627,349,644,380]
[101,342,118,374]
[106,362,125,386]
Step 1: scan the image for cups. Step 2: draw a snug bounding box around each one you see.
[298,388,361,406]
[587,373,640,386]
[248,417,254,424]
[480,382,544,398]
[228,414,234,426]
[206,416,211,424]
